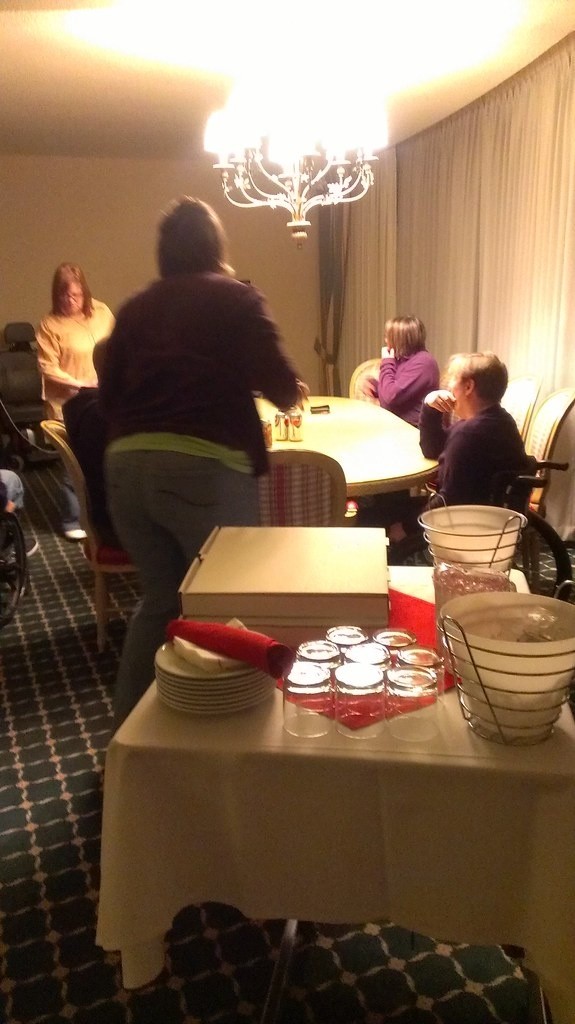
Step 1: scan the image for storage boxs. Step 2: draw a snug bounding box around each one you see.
[178,525,391,628]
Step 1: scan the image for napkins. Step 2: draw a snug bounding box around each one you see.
[165,619,297,680]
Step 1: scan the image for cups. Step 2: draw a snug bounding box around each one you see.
[335,663,387,738]
[283,661,334,738]
[296,640,342,691]
[385,665,444,743]
[372,628,417,667]
[345,642,392,672]
[325,624,370,665]
[396,645,444,704]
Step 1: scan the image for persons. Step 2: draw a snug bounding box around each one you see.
[0,468,39,558]
[35,261,117,541]
[351,352,524,564]
[63,338,110,554]
[380,315,441,505]
[102,195,308,734]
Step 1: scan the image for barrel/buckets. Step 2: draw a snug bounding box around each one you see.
[421,504,526,572]
[440,591,575,738]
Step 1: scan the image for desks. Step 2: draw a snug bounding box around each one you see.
[257,396,439,496]
[93,567,575,1024]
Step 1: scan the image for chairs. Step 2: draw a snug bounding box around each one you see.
[350,358,379,405]
[257,450,347,527]
[501,376,575,585]
[0,321,48,471]
[38,419,141,652]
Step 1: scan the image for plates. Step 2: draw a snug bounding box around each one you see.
[155,643,277,715]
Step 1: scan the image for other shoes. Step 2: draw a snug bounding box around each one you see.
[65,529,87,540]
[8,539,38,565]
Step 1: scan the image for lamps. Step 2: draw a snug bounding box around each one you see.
[200,60,390,250]
